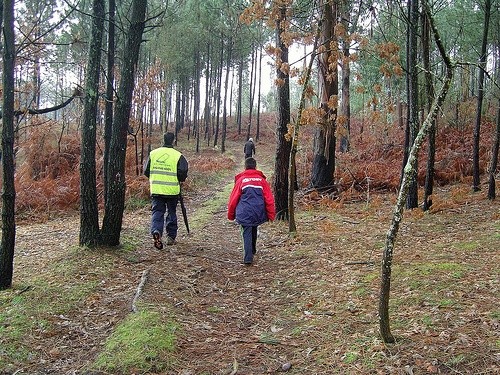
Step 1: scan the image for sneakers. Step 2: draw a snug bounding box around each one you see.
[166,236,177,245]
[153,230,163,250]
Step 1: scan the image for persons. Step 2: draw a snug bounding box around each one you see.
[243,137,256,167]
[142,132,188,250]
[228,158,275,265]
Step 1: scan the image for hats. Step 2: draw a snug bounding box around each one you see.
[249,138,253,142]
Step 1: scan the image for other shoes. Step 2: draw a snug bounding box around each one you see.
[244,261,252,264]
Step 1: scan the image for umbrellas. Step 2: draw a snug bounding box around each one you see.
[177,189,190,234]
